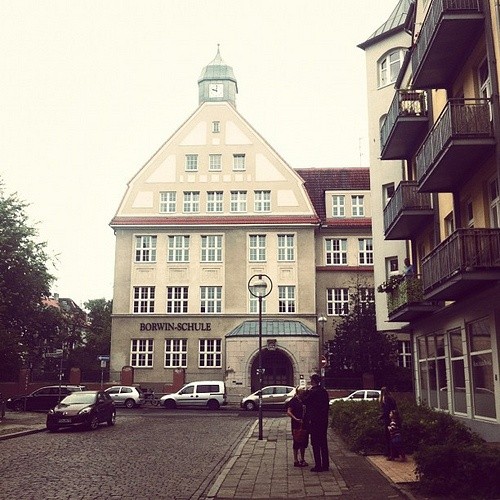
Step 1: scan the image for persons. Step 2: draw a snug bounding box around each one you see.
[305,373,330,472]
[288,383,310,468]
[380,386,402,461]
[401,258,418,280]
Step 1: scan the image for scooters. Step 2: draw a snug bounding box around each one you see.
[138,386,158,410]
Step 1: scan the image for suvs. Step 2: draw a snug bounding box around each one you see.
[328,390,386,406]
[47,390,116,433]
[440,386,493,393]
[7,385,90,414]
[100,384,145,409]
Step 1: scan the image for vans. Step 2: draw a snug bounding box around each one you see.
[161,380,226,411]
[241,386,301,409]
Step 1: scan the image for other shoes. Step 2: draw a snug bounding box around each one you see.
[300,460,308,466]
[322,466,329,471]
[310,466,322,472]
[294,461,303,467]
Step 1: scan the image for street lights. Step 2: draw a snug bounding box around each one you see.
[247,274,273,440]
